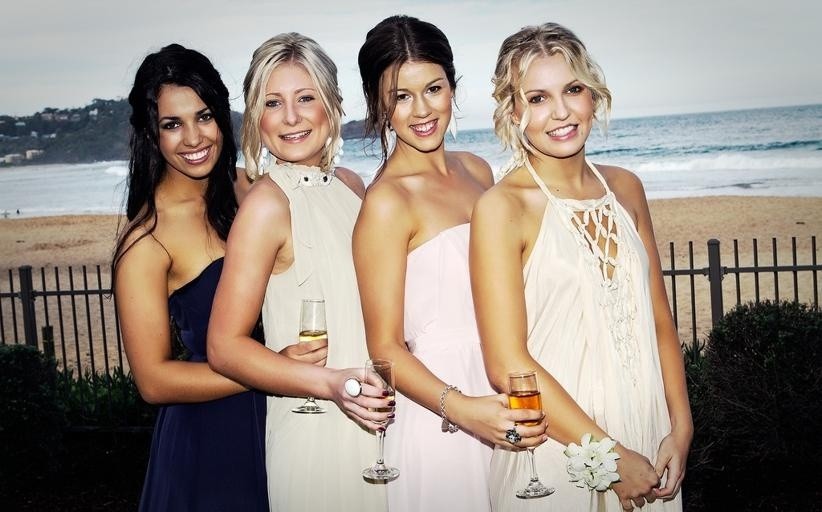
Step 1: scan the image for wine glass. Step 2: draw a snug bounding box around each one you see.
[363,358,401,485]
[290,298,328,413]
[506,370,555,499]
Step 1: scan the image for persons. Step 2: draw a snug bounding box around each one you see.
[351,15,550,512]
[105,43,331,511]
[203,29,397,512]
[469,20,702,512]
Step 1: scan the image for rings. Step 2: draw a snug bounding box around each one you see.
[512,423,518,431]
[503,430,523,446]
[345,376,362,396]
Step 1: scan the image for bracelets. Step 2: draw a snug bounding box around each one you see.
[439,385,462,433]
[562,432,621,494]
